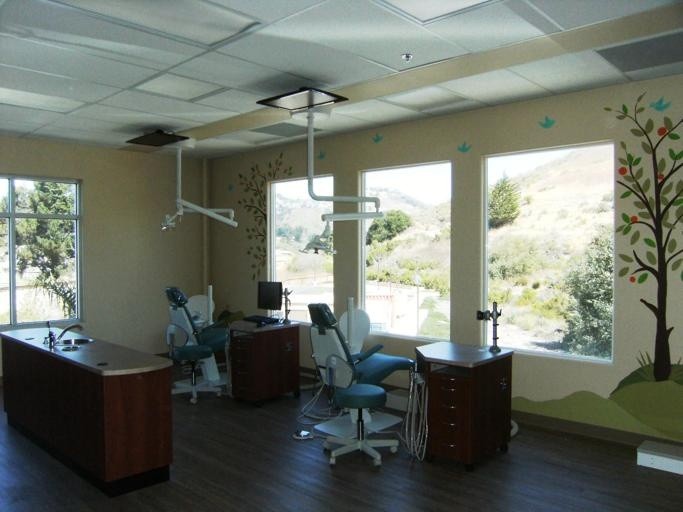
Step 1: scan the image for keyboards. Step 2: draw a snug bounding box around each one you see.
[244,315,279,324]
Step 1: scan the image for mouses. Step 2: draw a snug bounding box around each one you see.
[257,322,267,327]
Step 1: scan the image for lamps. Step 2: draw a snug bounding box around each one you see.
[125,130,190,147]
[256,86,350,112]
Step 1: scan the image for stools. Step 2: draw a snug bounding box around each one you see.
[323,383,400,467]
[172,345,221,404]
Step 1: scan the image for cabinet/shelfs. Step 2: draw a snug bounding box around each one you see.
[230,326,301,404]
[415,354,513,463]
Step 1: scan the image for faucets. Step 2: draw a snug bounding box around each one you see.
[49,324,83,349]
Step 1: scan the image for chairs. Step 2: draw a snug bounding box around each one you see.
[309,305,414,438]
[166,287,229,388]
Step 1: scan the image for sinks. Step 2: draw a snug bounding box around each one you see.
[55,338,94,347]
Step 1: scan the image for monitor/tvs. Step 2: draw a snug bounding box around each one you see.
[257,281,282,310]
